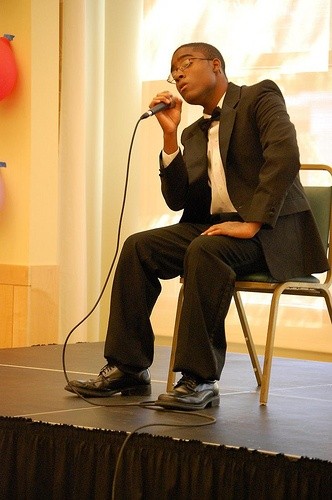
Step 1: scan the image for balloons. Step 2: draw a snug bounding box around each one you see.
[0,35,19,102]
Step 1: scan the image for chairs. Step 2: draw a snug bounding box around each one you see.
[166,164,332,406]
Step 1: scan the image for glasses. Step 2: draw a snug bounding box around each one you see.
[167,58,213,84]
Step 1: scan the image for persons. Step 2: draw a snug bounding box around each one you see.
[62,42,331,411]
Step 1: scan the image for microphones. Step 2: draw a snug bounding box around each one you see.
[140,96,176,119]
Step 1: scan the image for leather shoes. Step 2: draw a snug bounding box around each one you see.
[155,373,220,409]
[65,363,151,396]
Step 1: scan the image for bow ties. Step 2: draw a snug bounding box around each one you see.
[198,106,222,134]
